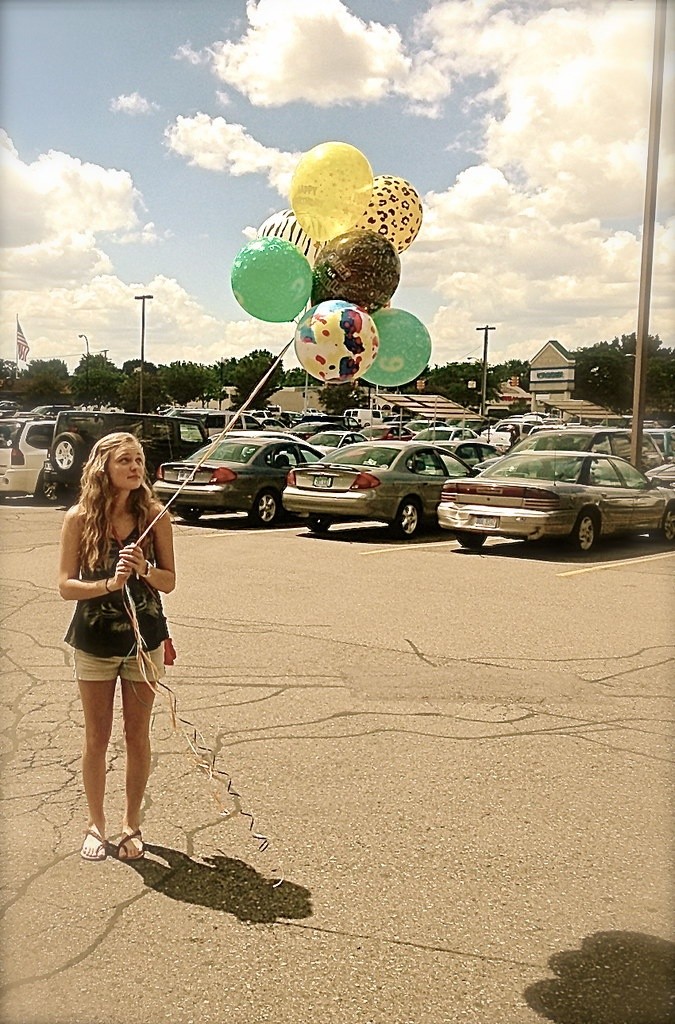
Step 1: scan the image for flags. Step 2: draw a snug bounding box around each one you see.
[17,319,29,362]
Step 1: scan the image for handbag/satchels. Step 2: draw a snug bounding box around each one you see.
[163,637,177,666]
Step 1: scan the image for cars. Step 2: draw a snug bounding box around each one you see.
[251,408,675,456]
[152,437,328,527]
[283,440,473,540]
[0,401,75,417]
[437,451,675,550]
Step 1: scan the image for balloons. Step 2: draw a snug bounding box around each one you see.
[347,175,425,256]
[231,236,313,322]
[257,208,331,271]
[294,300,380,384]
[311,229,401,315]
[291,142,374,247]
[360,308,432,387]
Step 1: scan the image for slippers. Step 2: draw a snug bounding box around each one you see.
[115,830,146,859]
[81,828,110,861]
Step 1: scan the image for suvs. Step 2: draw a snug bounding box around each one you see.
[0,417,69,504]
[47,411,211,507]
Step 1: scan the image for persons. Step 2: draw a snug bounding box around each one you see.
[508,423,521,447]
[58,431,175,860]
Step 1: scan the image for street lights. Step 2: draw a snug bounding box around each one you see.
[476,325,496,415]
[135,295,153,412]
[78,335,88,404]
[467,357,484,411]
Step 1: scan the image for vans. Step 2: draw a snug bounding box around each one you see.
[344,408,382,427]
[173,409,266,439]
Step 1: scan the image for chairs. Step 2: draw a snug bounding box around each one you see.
[412,460,425,473]
[537,469,555,478]
[276,454,290,469]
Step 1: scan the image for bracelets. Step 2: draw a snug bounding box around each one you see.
[139,560,153,578]
[105,578,112,592]
